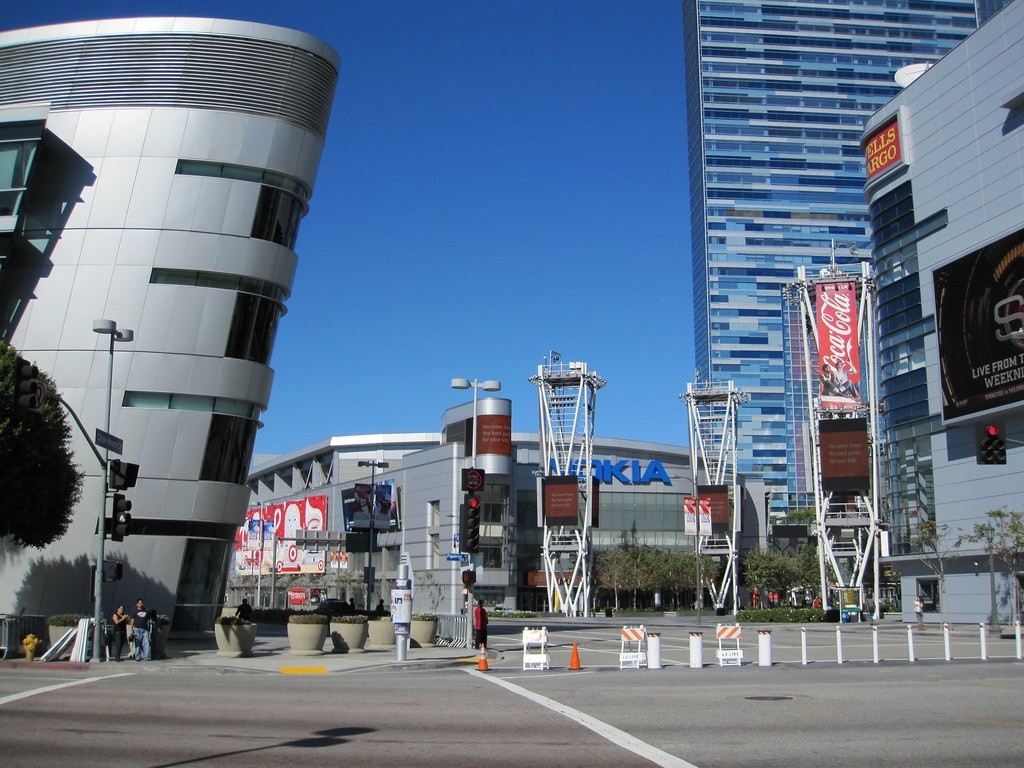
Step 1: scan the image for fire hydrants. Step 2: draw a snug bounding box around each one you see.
[22,633,43,661]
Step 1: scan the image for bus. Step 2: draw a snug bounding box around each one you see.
[786,582,901,607]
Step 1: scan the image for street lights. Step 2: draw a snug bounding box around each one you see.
[89,319,135,663]
[451,377,501,650]
[250,501,273,607]
[357,461,389,611]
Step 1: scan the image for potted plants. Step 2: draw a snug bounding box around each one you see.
[331,613,367,655]
[286,615,325,655]
[411,613,438,648]
[368,615,397,649]
[127,615,169,658]
[46,613,87,656]
[213,615,259,659]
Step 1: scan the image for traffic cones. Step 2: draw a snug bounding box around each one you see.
[568,642,583,671]
[476,642,493,671]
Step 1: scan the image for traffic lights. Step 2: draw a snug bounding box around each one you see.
[467,497,480,551]
[463,571,476,584]
[981,425,1006,462]
[117,462,139,487]
[116,499,133,536]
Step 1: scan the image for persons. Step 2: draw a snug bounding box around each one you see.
[349,598,356,612]
[235,598,252,620]
[819,357,861,401]
[827,595,833,610]
[474,600,488,650]
[913,595,925,630]
[376,598,384,612]
[865,594,876,625]
[813,596,822,608]
[112,605,128,662]
[129,599,154,661]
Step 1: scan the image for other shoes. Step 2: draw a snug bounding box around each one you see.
[116,658,124,662]
